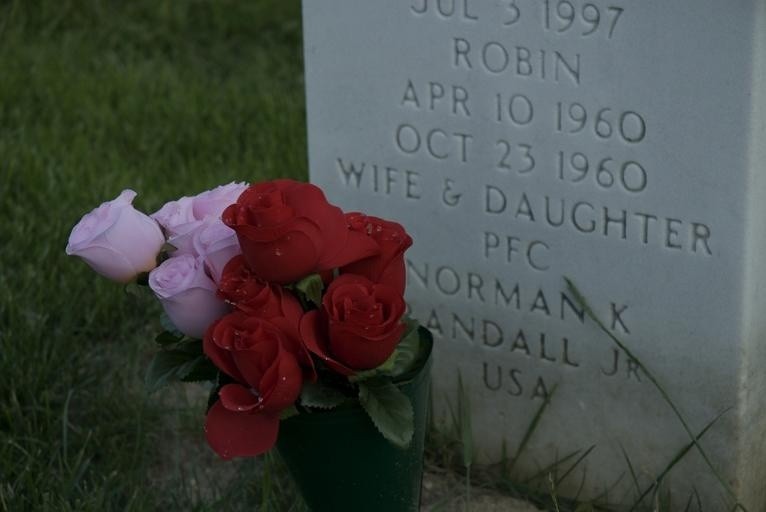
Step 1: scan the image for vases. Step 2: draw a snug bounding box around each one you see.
[65,177,417,463]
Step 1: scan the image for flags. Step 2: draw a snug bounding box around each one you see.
[274,327,434,512]
